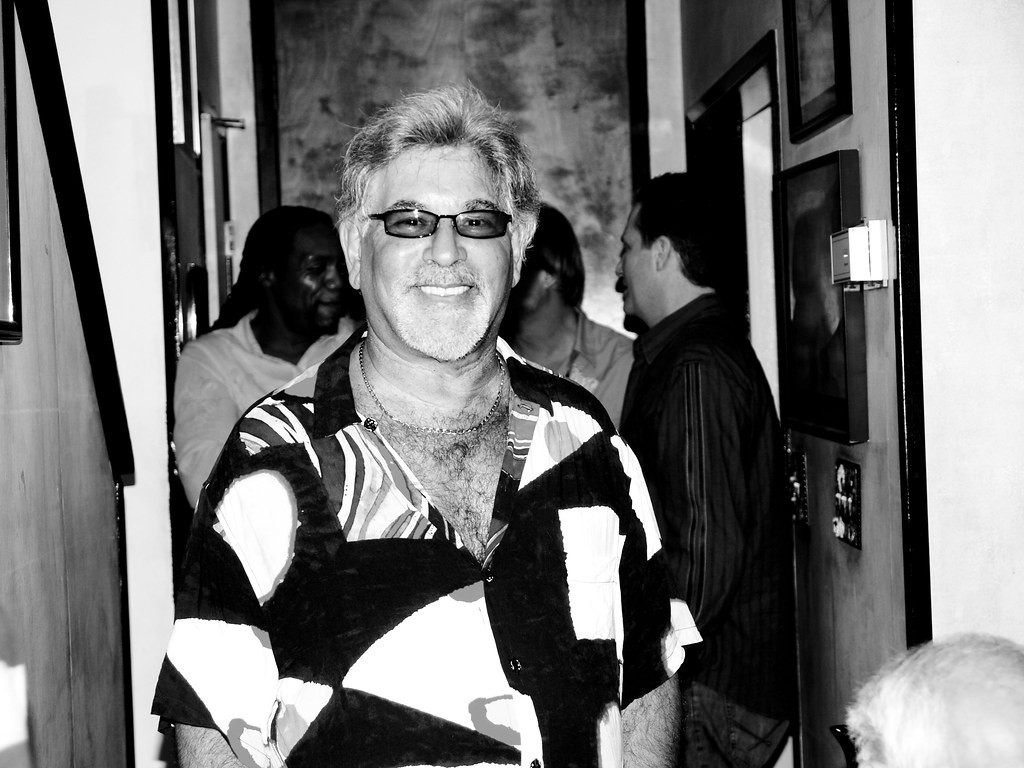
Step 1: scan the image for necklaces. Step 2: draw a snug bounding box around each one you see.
[358,341,505,436]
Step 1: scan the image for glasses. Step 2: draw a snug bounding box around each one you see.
[367,206,515,242]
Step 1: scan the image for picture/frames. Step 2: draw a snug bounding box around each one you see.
[0,0,23,344]
[781,0,854,143]
[771,148,870,446]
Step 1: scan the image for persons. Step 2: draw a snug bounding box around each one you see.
[172,205,355,508]
[838,635,1024,768]
[152,85,704,768]
[500,204,634,430]
[613,171,800,768]
